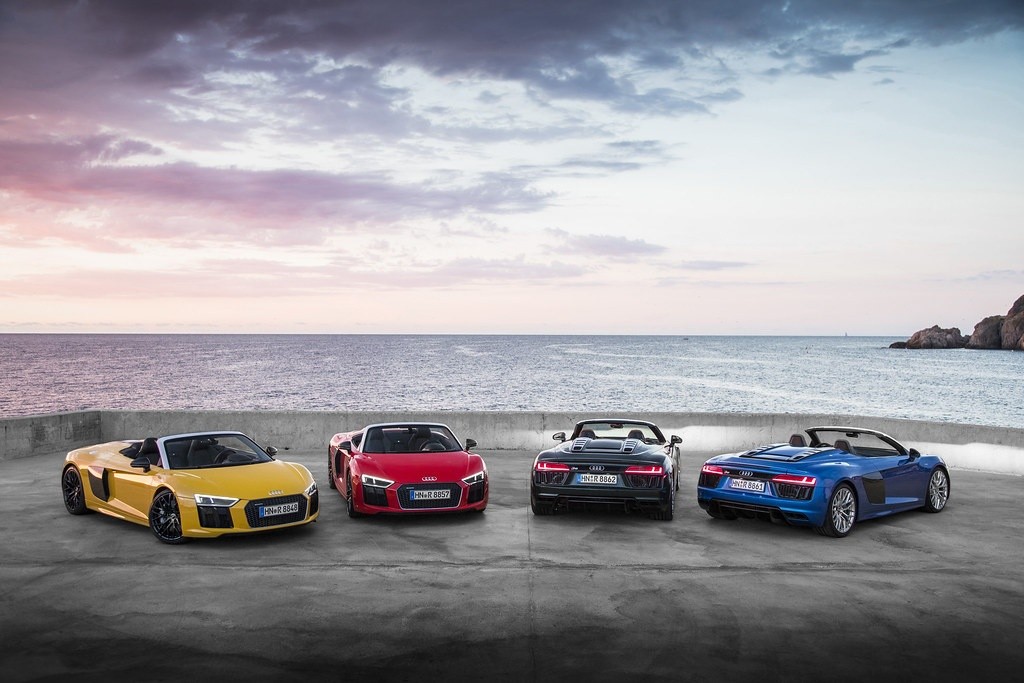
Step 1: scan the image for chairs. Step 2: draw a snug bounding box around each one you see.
[834,440,857,455]
[408,427,433,452]
[580,429,596,440]
[187,438,220,466]
[628,430,646,445]
[789,433,808,448]
[135,438,161,466]
[364,429,390,453]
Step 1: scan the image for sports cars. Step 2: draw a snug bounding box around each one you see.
[328,422,499,518]
[61,431,318,545]
[530,418,683,522]
[697,426,952,538]
